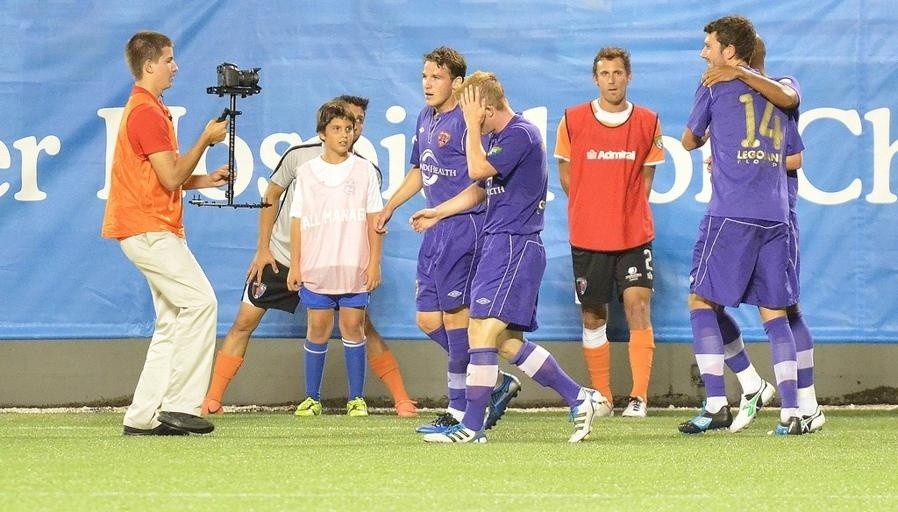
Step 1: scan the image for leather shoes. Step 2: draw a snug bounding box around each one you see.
[123,423,189,436]
[160,411,214,435]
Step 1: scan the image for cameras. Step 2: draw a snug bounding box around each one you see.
[217,62,263,89]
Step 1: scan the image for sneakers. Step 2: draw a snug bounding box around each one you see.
[394,400,418,417]
[346,395,368,417]
[417,371,649,443]
[201,398,223,416]
[676,379,827,435]
[294,396,323,417]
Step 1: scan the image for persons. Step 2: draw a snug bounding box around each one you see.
[286,99,384,418]
[407,68,614,448]
[674,12,805,439]
[201,94,422,422]
[699,31,827,432]
[373,45,525,436]
[97,30,240,436]
[552,46,666,421]
